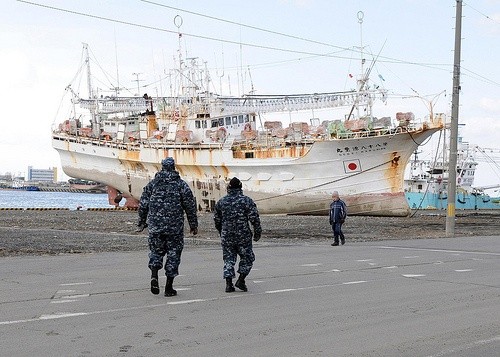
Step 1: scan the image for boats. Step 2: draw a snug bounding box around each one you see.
[48,35,446,216]
[404,135,498,209]
[67,177,108,193]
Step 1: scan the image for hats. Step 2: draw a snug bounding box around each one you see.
[228,176,242,189]
[331,191,339,197]
[160,156,176,172]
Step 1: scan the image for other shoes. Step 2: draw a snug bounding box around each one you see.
[340,238,346,245]
[330,241,340,246]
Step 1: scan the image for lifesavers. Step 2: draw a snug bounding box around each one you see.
[244,123,251,132]
[218,127,226,138]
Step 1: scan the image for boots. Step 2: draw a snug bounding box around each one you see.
[164,277,178,296]
[151,270,160,295]
[225,277,236,293]
[234,273,248,291]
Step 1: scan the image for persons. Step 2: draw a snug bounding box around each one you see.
[213,176,262,293]
[137,156,198,297]
[328,191,347,246]
